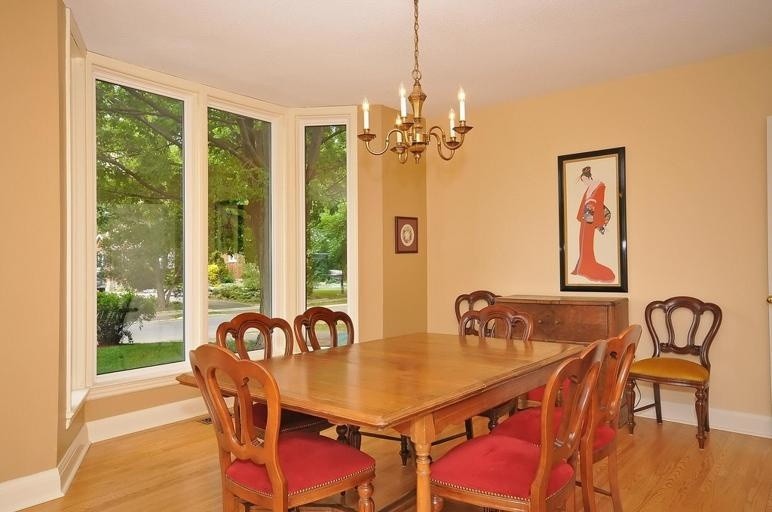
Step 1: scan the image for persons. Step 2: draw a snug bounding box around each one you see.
[570,166,615,283]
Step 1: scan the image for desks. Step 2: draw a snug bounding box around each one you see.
[175,334,585,512]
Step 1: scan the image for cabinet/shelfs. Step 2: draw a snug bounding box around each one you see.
[495,295,631,428]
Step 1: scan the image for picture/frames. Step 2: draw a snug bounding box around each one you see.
[395,216,418,254]
[558,147,627,293]
[214,201,243,259]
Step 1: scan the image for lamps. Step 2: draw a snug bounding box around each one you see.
[357,0,474,164]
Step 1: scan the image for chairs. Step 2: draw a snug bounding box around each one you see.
[430,333,607,512]
[455,290,502,335]
[294,301,359,352]
[217,313,349,444]
[461,304,533,430]
[631,296,723,448]
[182,339,375,512]
[489,323,643,512]
